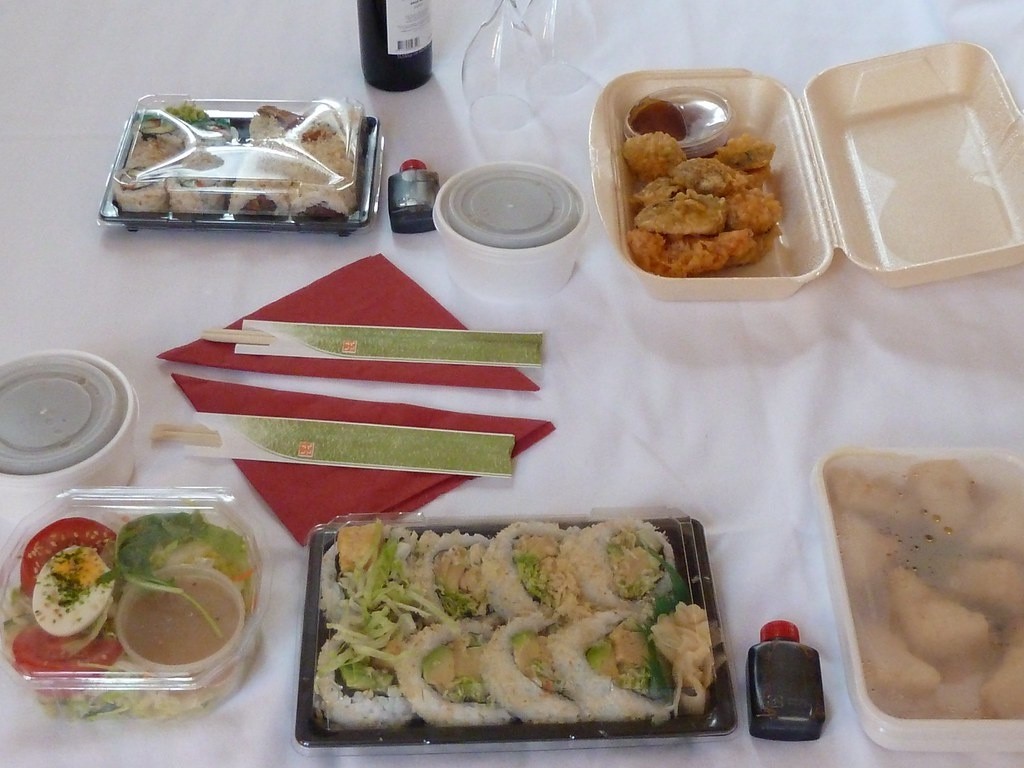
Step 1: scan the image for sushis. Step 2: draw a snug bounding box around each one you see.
[314,519,686,733]
[112,106,357,222]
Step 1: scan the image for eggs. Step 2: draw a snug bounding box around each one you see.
[32,545,113,635]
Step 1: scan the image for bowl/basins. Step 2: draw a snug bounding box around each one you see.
[624,88,731,159]
[291,508,742,754]
[1,348,139,527]
[98,94,384,235]
[434,163,588,304]
[587,43,1023,301]
[2,487,273,732]
[817,446,1024,756]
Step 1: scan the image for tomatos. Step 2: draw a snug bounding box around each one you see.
[12,624,122,693]
[20,516,117,594]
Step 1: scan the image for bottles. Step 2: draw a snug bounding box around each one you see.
[387,160,440,233]
[745,620,826,741]
[357,0,432,92]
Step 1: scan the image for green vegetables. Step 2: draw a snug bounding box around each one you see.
[43,512,229,638]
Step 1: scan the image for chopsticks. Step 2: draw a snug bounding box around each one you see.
[157,409,516,479]
[200,326,545,368]
